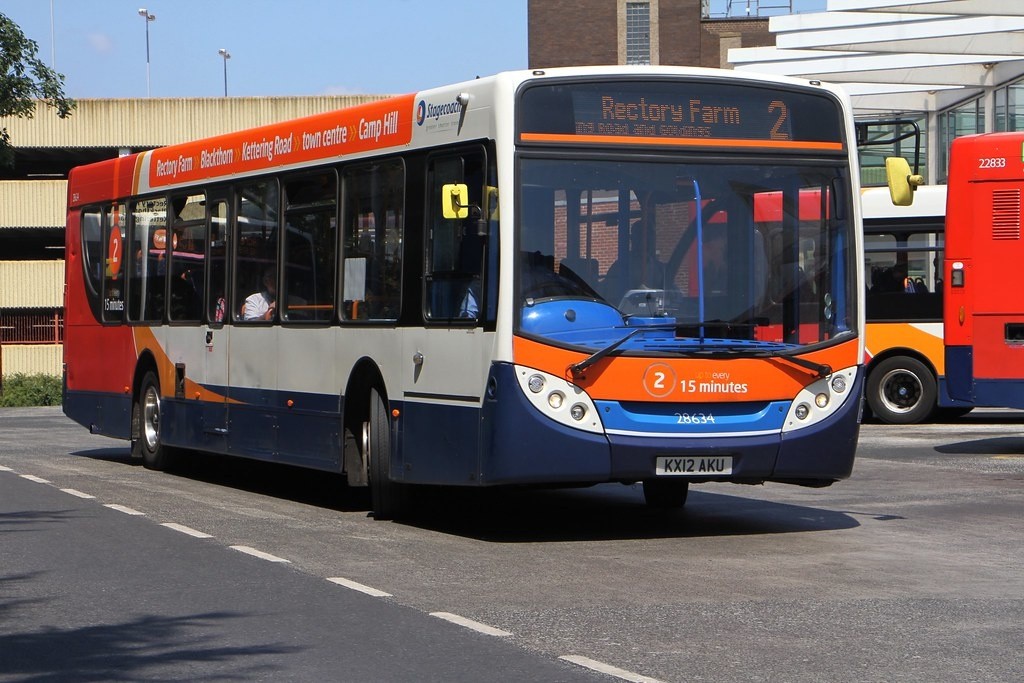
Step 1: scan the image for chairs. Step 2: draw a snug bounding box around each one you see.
[241,304,245,320]
[915,278,930,299]
[266,308,273,321]
[871,264,889,289]
[904,276,917,297]
[559,258,599,283]
[445,236,482,318]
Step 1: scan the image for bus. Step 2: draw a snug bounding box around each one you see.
[943,130,1024,410]
[686,184,948,425]
[61,64,925,523]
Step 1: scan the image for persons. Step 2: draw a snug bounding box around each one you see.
[244,270,307,321]
[604,221,682,306]
[461,222,565,319]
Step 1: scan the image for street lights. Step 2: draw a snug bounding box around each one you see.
[219,48,231,97]
[138,8,155,99]
